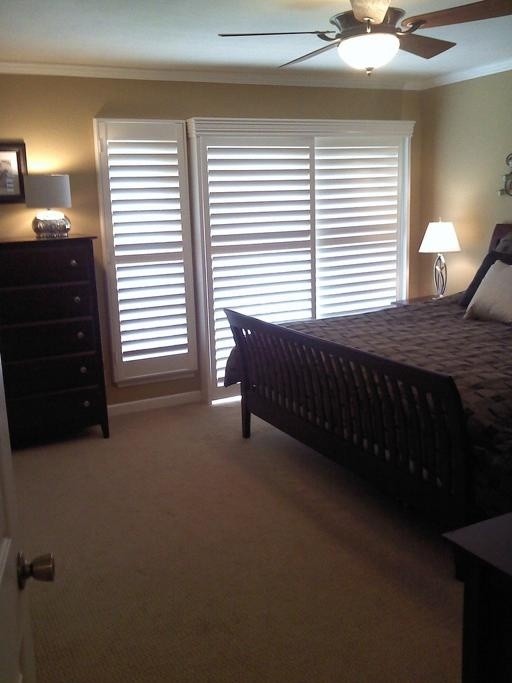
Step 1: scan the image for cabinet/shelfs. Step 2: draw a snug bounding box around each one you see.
[440,511,512,683]
[0,232,111,453]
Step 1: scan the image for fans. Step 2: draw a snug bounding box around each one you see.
[217,0,512,70]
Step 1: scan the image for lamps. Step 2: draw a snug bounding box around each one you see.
[28,174,75,239]
[417,221,464,300]
[329,7,405,79]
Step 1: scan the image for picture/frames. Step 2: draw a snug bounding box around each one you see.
[0,142,32,206]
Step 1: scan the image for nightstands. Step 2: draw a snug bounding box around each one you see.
[391,295,442,306]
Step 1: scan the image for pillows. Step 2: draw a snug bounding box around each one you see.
[461,238,512,326]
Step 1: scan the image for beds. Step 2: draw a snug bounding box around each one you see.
[223,222,512,579]
